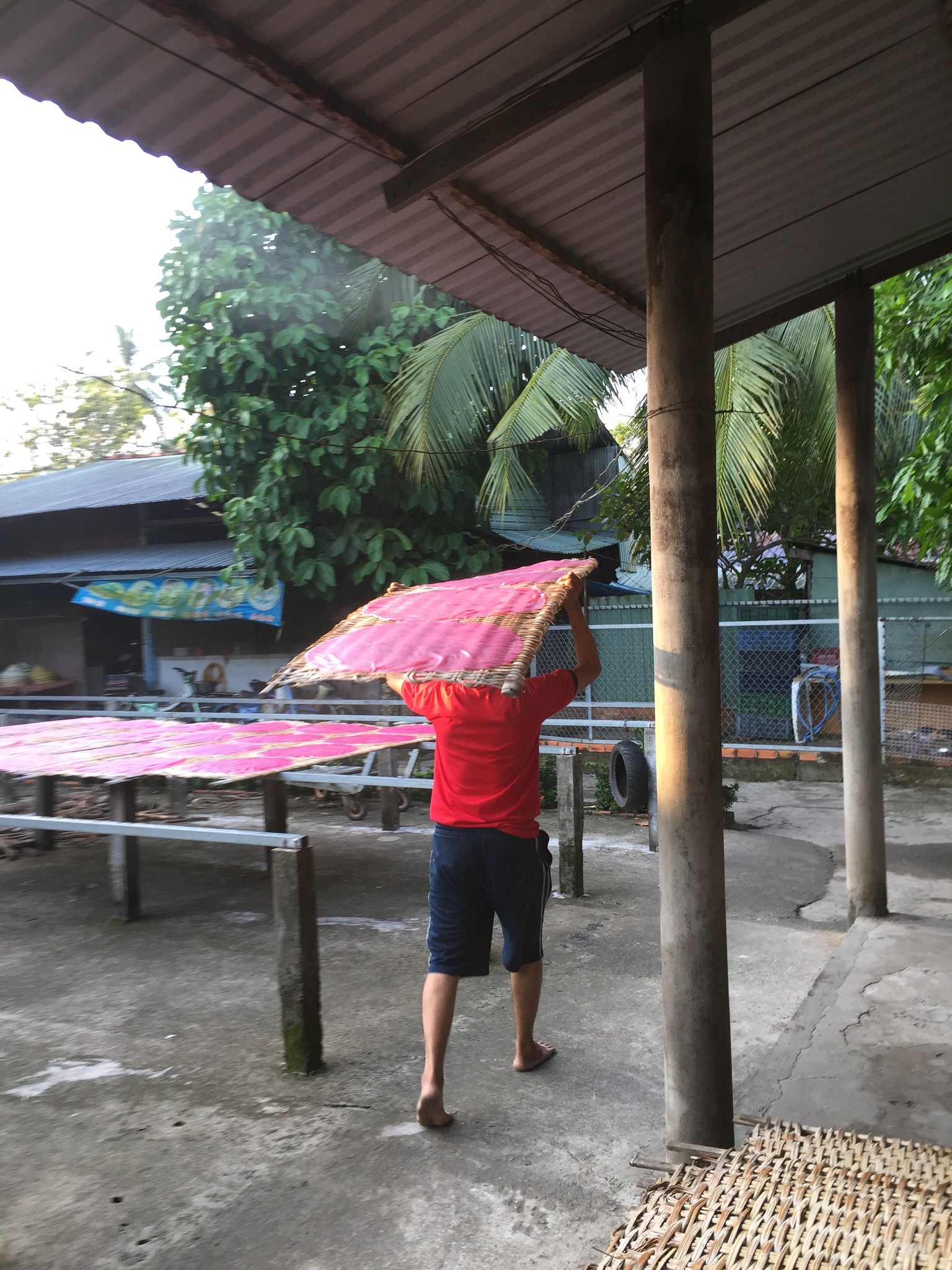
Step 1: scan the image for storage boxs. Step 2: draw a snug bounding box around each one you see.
[735,605,802,740]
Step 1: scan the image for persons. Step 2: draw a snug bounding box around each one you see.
[387,573,601,1127]
